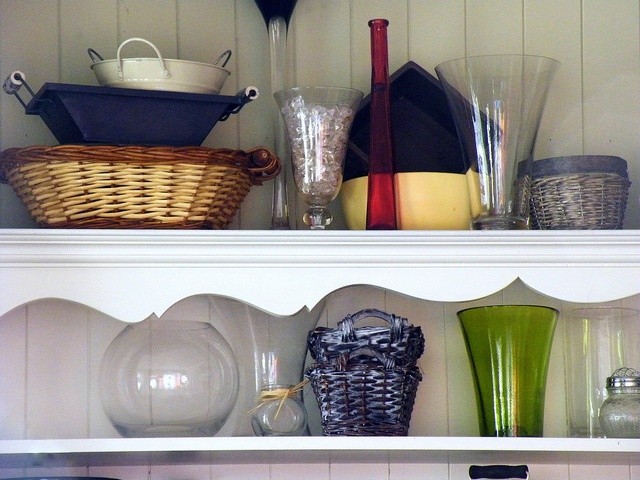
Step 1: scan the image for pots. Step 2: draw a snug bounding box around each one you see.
[88,37,231,94]
[4,71,260,146]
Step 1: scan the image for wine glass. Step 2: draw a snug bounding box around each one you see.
[272,88,364,230]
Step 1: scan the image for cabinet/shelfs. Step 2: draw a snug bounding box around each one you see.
[0,229,640,454]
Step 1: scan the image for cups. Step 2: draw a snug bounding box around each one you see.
[435,54,559,229]
[209,290,326,438]
[567,309,640,437]
[456,305,559,438]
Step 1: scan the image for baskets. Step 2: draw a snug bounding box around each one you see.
[303,349,422,436]
[0,145,281,230]
[532,172,633,229]
[307,309,424,367]
